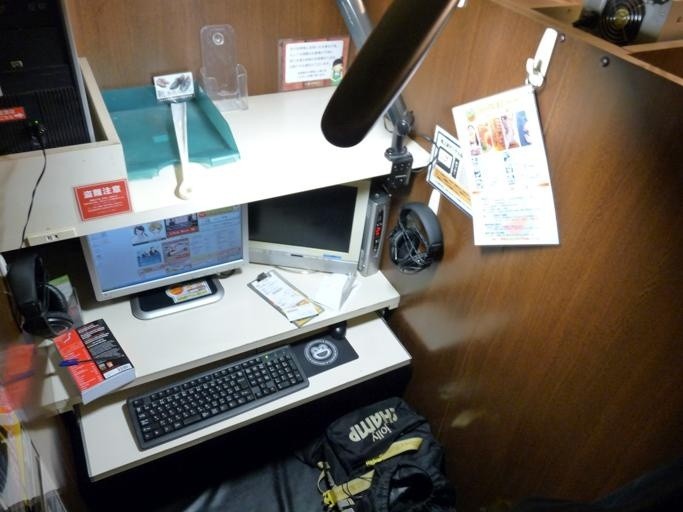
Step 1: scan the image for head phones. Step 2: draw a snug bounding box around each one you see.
[6,247,75,339]
[386,207,445,274]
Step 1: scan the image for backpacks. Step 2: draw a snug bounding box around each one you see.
[319,397,448,512]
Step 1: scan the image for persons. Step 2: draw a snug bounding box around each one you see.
[132,211,194,264]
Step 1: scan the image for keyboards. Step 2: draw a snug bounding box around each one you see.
[122,346,309,451]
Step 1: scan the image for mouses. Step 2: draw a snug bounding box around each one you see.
[330,320,347,340]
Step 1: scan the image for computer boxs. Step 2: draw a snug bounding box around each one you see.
[578,1,671,46]
[0,0,96,157]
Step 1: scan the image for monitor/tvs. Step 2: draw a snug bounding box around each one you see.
[80,205,249,320]
[247,180,371,275]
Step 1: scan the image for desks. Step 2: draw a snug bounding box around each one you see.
[1,82,433,511]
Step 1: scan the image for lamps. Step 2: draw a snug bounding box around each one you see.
[321,0,458,196]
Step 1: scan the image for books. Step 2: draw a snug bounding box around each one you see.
[50,316,136,406]
